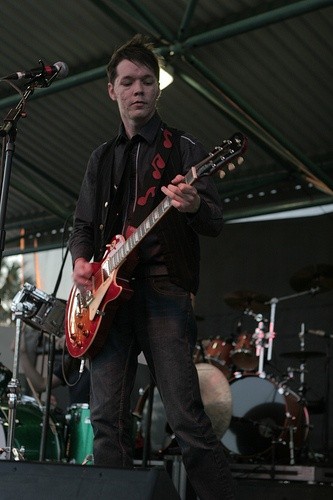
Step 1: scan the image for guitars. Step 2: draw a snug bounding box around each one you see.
[65,131,250,359]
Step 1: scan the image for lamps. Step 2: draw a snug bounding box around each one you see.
[159,65,175,91]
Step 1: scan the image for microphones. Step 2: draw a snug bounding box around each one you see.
[5,61,70,83]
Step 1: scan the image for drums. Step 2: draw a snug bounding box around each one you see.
[0,392,63,462]
[230,332,260,371]
[193,335,231,378]
[220,372,311,464]
[145,363,230,455]
[9,283,66,338]
[63,403,136,465]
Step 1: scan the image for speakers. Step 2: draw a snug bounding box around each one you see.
[0,459,184,500]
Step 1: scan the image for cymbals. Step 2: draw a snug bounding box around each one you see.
[224,289,271,313]
[289,263,333,294]
[279,348,326,362]
[278,328,326,340]
[192,308,208,321]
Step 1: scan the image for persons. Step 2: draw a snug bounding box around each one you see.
[11,332,90,463]
[70,42,239,500]
